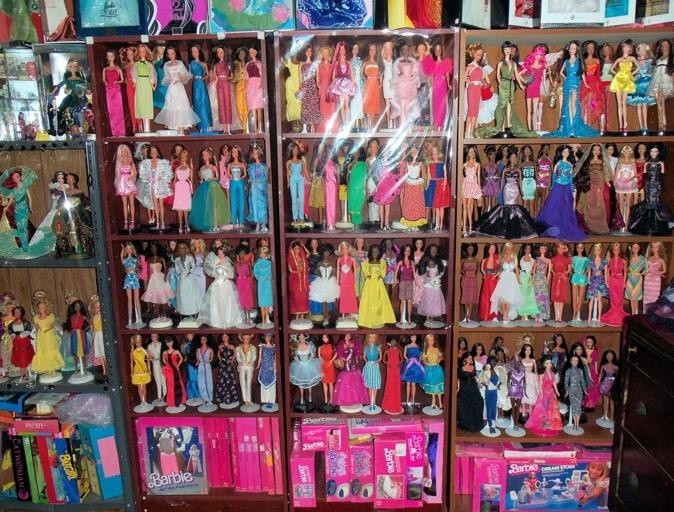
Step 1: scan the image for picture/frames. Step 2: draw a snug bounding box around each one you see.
[73,1,674,35]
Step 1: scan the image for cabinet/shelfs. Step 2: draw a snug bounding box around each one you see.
[273,27,458,512]
[606,311,673,512]
[452,32,674,512]
[0,37,126,510]
[86,35,287,511]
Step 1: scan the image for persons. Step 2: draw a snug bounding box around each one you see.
[285,138,451,233]
[462,143,673,241]
[113,140,268,235]
[518,471,539,505]
[463,38,674,139]
[577,462,610,506]
[456,332,620,438]
[0,165,96,261]
[0,288,107,388]
[458,241,667,329]
[281,39,453,135]
[288,237,447,331]
[129,332,280,413]
[288,332,445,416]
[102,41,264,137]
[119,237,274,330]
[0,42,95,141]
